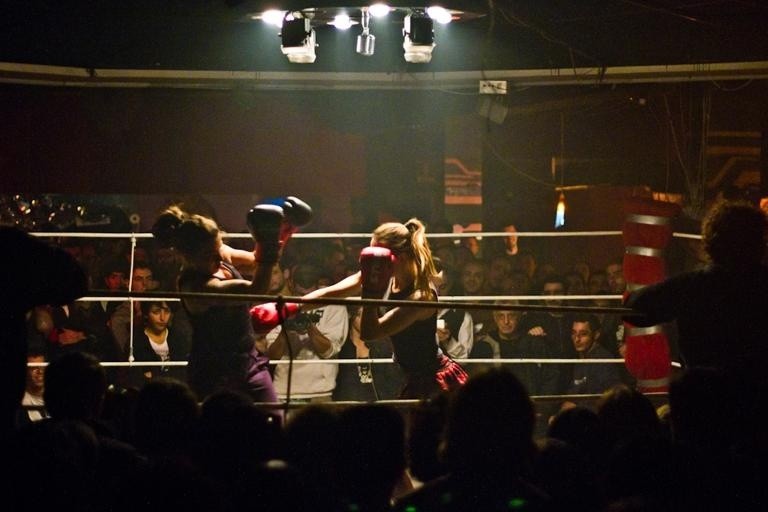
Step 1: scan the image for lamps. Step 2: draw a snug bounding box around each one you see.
[247,4,487,65]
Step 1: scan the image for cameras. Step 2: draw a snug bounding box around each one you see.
[284,311,319,334]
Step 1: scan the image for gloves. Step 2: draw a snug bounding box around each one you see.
[246,196,312,264]
[359,246,397,299]
[250,300,301,334]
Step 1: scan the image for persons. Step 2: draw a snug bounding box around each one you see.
[2,192,768,512]
